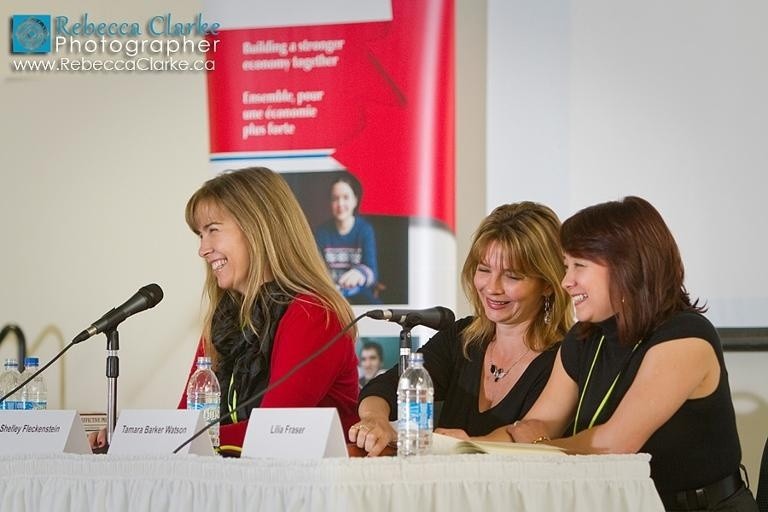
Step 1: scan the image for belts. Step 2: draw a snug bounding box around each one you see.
[683,474,743,511]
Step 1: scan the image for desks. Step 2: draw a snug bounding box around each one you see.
[0,450,665,512]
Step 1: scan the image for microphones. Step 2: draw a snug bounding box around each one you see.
[365,306,457,331]
[72,283,164,342]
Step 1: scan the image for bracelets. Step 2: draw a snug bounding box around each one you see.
[532,435,549,444]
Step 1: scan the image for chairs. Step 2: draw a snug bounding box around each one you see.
[721,350,768,502]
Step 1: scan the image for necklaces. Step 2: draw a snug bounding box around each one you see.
[490,339,531,383]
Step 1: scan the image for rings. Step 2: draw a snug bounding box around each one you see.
[350,425,359,430]
[360,425,369,431]
[513,420,521,426]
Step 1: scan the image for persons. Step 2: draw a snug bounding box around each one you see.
[348,201,572,457]
[432,196,762,512]
[179,167,358,447]
[314,174,384,305]
[357,343,390,391]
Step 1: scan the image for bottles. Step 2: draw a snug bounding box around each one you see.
[396,350,434,454]
[2,357,25,411]
[186,356,220,455]
[24,356,47,405]
